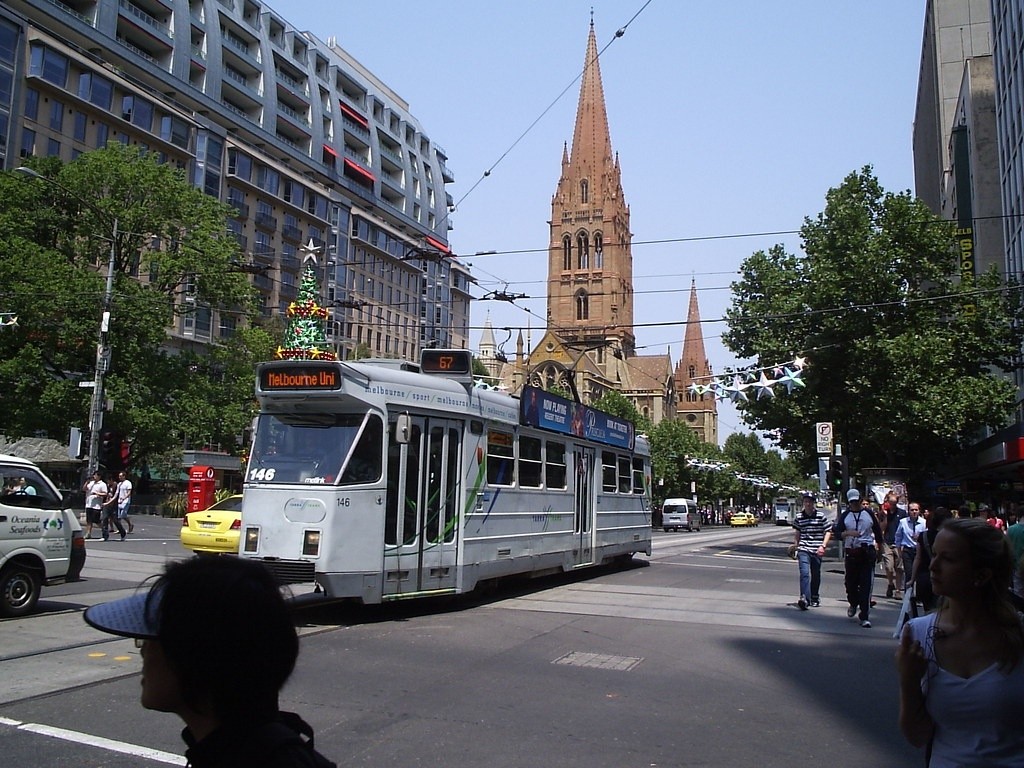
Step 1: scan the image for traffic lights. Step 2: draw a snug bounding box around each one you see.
[828,456,849,491]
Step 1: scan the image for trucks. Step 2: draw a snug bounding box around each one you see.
[0,454,87,616]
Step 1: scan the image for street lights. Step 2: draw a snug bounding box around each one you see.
[16,166,118,477]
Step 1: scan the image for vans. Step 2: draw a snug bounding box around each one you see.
[661,498,701,532]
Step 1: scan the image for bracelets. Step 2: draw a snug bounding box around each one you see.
[820,544,827,549]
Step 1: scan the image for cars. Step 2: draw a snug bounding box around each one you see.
[817,502,823,508]
[730,512,760,528]
[180,493,243,556]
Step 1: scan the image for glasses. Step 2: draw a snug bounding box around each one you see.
[134,637,152,649]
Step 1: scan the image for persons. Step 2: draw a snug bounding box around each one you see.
[896,518,1024,768]
[572,411,585,437]
[82,554,338,768]
[749,504,771,520]
[788,491,833,611]
[833,489,884,627]
[859,491,1024,619]
[0,477,37,496]
[82,470,135,542]
[525,390,539,429]
[698,509,716,525]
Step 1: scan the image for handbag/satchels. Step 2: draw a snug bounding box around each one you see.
[894,585,917,640]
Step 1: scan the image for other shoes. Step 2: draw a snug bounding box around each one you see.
[860,619,871,627]
[848,604,857,617]
[798,598,820,611]
[886,584,902,600]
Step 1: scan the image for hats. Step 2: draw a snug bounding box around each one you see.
[802,492,816,501]
[847,489,860,502]
[83,582,164,638]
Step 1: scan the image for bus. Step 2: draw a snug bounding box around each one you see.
[774,496,797,526]
[236,347,654,606]
